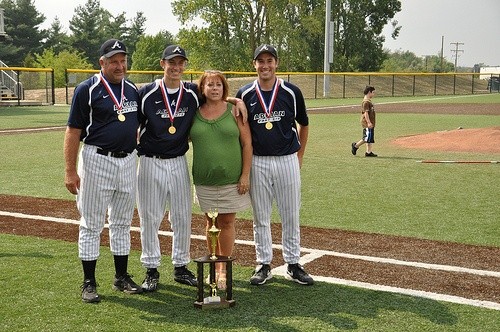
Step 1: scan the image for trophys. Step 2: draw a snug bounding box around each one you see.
[193,208,236,310]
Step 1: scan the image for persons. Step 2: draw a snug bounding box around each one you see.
[135,44,249,293]
[352,85,377,157]
[189,70,249,288]
[236,44,314,286]
[64,39,143,302]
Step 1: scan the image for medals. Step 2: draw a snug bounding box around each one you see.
[265,122,273,129]
[168,126,176,134]
[118,115,125,122]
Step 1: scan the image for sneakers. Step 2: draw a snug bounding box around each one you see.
[365,152,377,157]
[80,279,100,303]
[141,271,160,292]
[113,274,142,294]
[285,264,313,285]
[351,142,358,155]
[250,264,273,285]
[173,268,203,287]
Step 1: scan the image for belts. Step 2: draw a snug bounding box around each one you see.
[144,154,177,159]
[96,146,135,158]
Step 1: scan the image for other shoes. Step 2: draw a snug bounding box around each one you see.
[218,276,227,291]
[205,272,219,284]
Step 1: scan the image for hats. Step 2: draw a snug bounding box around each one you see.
[100,39,130,58]
[254,44,277,60]
[162,45,189,61]
